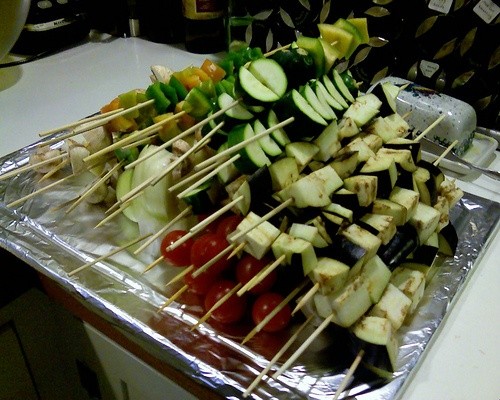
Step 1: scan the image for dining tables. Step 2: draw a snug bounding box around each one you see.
[1,36,500,400]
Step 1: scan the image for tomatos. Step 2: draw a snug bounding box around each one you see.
[162,216,291,333]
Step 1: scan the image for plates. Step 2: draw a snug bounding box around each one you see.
[418,130,498,176]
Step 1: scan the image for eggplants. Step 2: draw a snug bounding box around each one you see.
[223,84,464,387]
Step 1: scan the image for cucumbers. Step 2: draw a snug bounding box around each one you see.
[182,18,371,211]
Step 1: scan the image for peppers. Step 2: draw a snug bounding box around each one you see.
[98,44,266,166]
